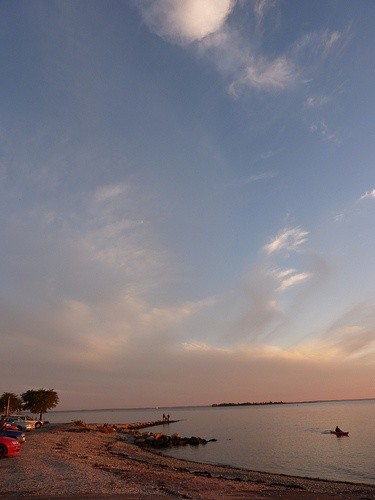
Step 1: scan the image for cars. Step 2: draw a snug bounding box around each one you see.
[0,436,21,458]
[0,424,26,444]
[0,414,45,432]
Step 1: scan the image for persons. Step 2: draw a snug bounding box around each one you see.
[163,413,170,421]
[335,426,342,432]
[0,417,9,436]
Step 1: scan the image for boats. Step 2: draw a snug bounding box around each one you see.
[330,429,349,436]
